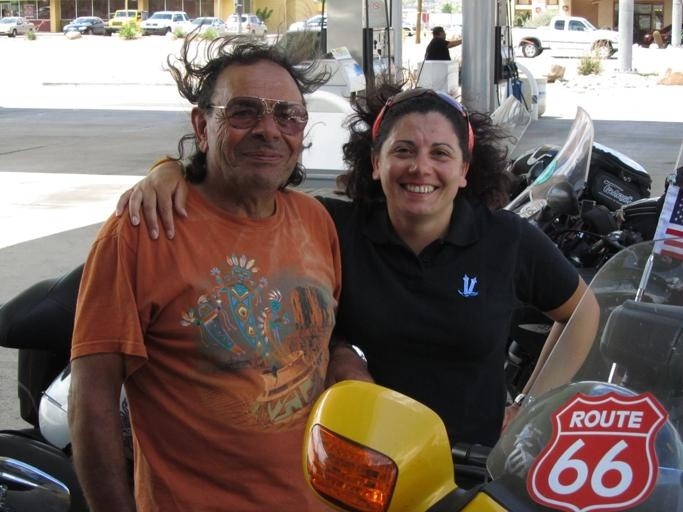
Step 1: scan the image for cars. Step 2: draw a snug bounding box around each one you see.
[63,16,109,35]
[289,10,328,32]
[0,17,36,38]
[643,22,683,49]
[182,16,225,38]
[30,5,50,18]
[402,8,462,37]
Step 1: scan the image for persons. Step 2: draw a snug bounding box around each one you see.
[115,85,600,512]
[425,26,462,60]
[69,36,342,512]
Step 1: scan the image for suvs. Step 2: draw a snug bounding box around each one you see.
[140,11,190,37]
[224,13,268,42]
[103,10,149,38]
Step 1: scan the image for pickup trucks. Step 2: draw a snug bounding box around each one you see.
[510,16,619,59]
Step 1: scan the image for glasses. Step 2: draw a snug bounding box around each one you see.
[210,95,309,137]
[371,89,476,158]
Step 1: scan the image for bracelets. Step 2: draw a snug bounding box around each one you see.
[513,393,538,412]
